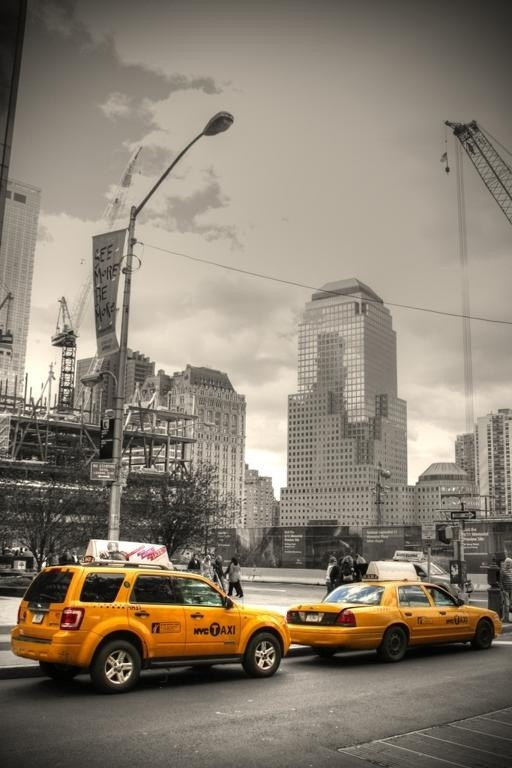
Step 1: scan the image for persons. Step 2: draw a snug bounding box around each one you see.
[498,557,512,624]
[202,554,215,581]
[188,552,201,604]
[215,554,227,596]
[321,552,369,601]
[226,558,244,599]
[3,546,79,567]
[224,557,240,596]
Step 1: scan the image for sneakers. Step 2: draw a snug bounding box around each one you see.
[226,592,243,598]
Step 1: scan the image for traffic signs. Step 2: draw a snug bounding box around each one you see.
[89,460,119,482]
[450,510,478,520]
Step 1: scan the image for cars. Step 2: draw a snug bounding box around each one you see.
[391,548,475,601]
[283,557,508,663]
[9,535,297,692]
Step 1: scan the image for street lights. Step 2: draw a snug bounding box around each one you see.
[79,109,237,538]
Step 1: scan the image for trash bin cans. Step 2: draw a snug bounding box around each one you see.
[487,588,512,625]
